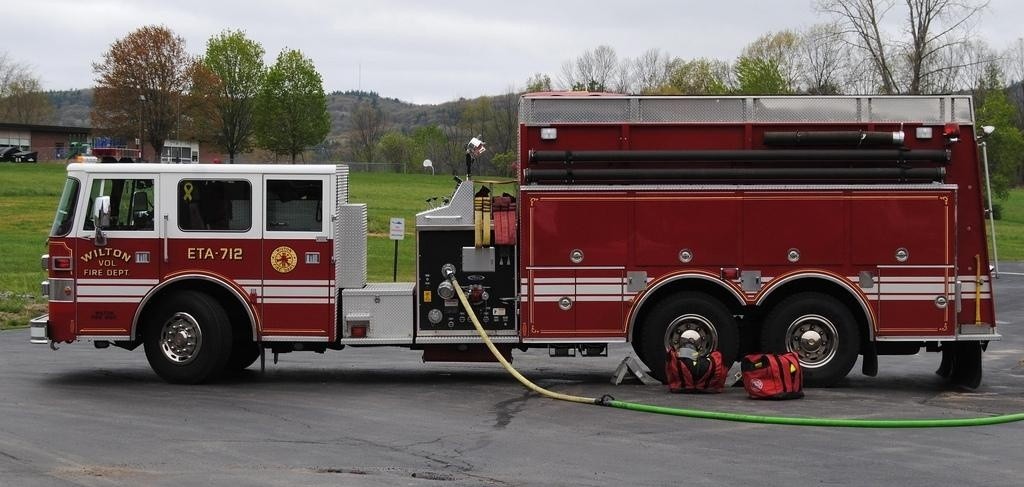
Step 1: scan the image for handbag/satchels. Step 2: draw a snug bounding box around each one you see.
[741,351,806,400]
[665,347,728,394]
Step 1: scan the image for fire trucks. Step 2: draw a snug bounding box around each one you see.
[29,91,1002,389]
